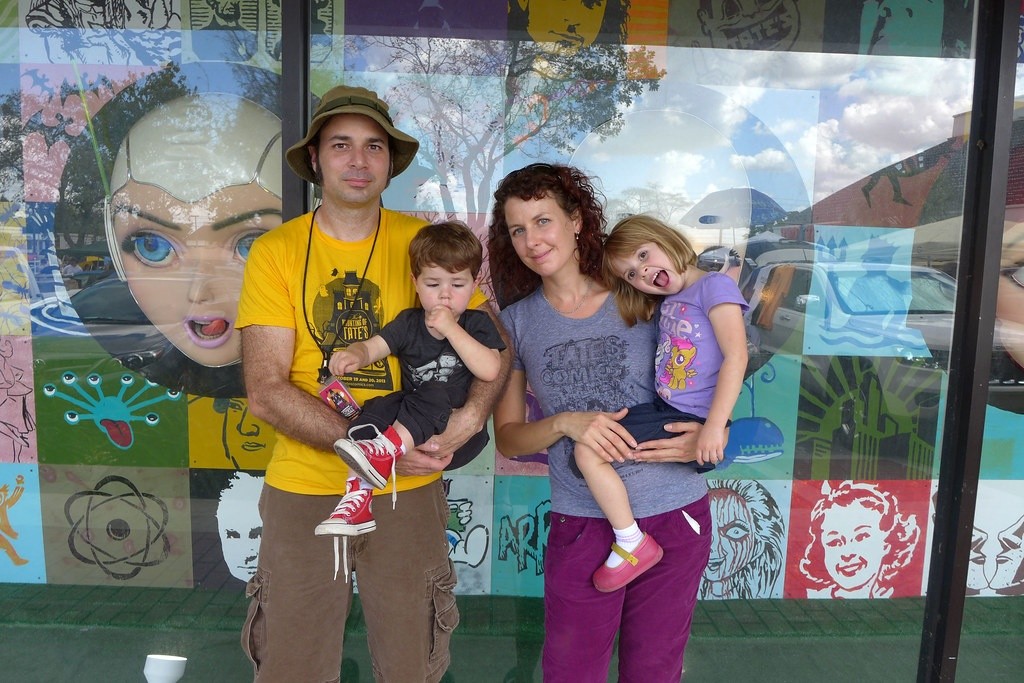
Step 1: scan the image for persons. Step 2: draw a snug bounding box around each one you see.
[234,85,516,683]
[314,221,508,585]
[486,163,731,683]
[100,89,313,369]
[574,216,750,592]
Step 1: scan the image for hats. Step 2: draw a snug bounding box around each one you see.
[285,85,419,183]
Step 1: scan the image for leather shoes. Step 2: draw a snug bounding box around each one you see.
[593,532,663,592]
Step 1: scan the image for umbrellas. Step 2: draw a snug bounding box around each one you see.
[680,187,787,245]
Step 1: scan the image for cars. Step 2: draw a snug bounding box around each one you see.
[0,246,51,336]
[696,238,1024,458]
[0,261,242,420]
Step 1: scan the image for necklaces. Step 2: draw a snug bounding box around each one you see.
[538,279,593,314]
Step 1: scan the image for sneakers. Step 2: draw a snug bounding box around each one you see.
[314,477,376,584]
[333,424,407,510]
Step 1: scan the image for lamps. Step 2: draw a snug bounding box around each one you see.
[143,654,187,683]
